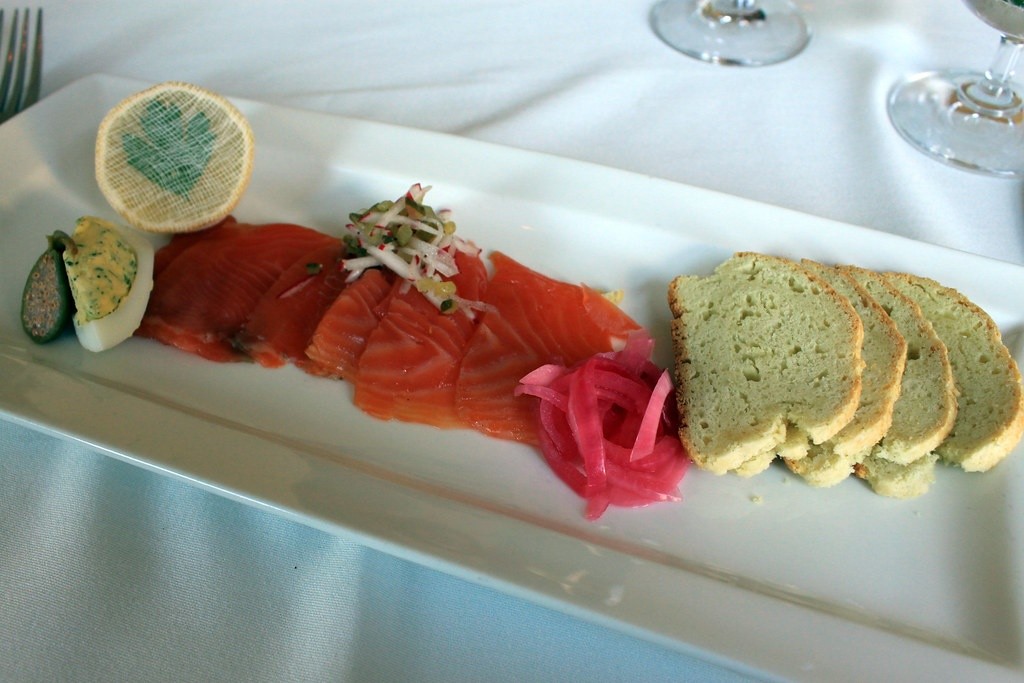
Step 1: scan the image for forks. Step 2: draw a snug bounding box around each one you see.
[0,7,50,123]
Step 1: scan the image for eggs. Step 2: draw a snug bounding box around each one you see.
[63,215,156,353]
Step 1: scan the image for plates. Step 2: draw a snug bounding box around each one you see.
[0,75,1024,683]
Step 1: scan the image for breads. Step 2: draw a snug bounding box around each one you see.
[669,250,1023,496]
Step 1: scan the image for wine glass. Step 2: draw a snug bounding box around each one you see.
[649,0,808,65]
[886,1,1024,176]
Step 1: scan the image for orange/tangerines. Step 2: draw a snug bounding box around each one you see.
[94,82,253,234]
